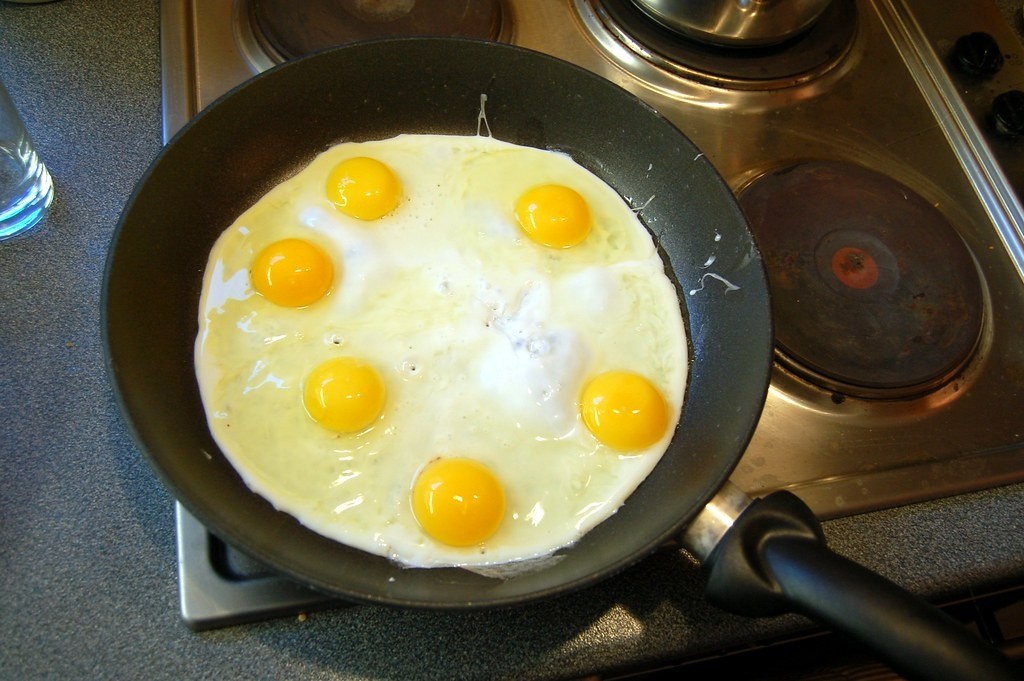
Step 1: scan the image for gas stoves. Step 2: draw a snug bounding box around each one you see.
[170,0,1024,635]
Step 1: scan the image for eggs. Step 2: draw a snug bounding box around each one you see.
[194,135,689,565]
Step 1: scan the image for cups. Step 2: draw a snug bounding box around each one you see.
[0,79,54,242]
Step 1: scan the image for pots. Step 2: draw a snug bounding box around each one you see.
[98,33,1024,681]
